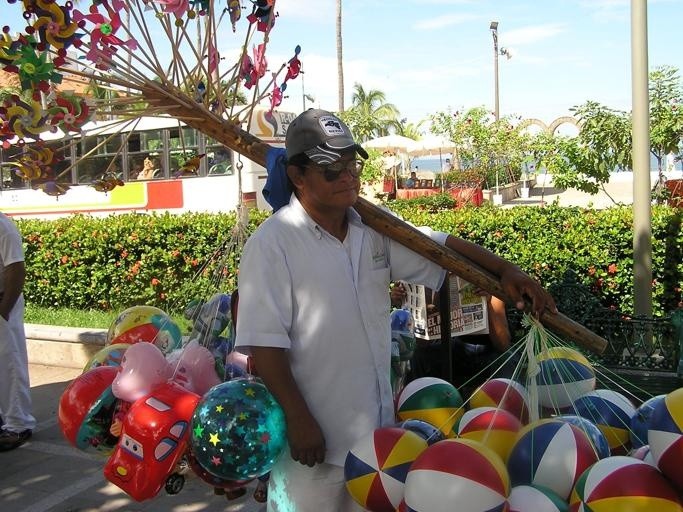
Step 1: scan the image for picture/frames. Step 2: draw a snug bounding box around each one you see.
[412,176,451,190]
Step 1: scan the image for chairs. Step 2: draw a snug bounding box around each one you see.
[58,157,236,184]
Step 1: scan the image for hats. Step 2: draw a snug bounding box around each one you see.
[285,108,369,165]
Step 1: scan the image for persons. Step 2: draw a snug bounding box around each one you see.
[233,105,554,512]
[405,172,418,188]
[131,148,231,180]
[1,211,34,458]
[441,158,453,176]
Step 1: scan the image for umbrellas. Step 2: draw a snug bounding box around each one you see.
[359,133,418,191]
[414,132,458,188]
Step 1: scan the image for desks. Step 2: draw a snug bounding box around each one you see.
[395,185,485,211]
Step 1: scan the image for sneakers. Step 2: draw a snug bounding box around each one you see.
[0,430,32,452]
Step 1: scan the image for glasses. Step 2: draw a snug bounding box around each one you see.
[295,158,364,182]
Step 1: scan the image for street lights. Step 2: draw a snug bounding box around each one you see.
[303,92,315,111]
[489,22,512,122]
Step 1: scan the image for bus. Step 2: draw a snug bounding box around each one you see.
[1,107,299,221]
